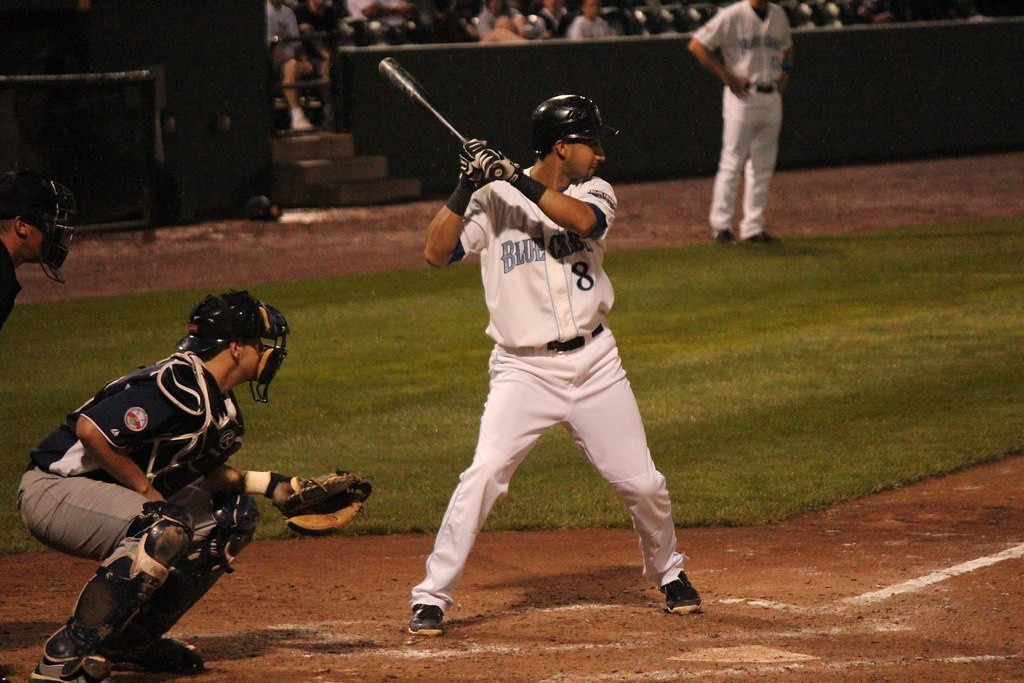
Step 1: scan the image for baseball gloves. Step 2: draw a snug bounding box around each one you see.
[287,472,371,531]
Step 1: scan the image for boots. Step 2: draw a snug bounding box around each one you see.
[97,493,260,676]
[29,501,195,683]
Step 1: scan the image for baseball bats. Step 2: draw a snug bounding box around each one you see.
[378,55,503,182]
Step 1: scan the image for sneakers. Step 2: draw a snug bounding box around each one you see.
[407,603,445,636]
[659,571,701,614]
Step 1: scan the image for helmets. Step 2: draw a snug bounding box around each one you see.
[530,95,619,160]
[2,168,56,223]
[177,292,262,355]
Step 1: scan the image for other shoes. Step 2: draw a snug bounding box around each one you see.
[713,227,778,246]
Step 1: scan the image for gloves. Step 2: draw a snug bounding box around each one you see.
[473,148,523,185]
[457,137,487,185]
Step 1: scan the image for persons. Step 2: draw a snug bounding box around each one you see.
[402,94,703,640]
[13,287,373,683]
[687,1,796,245]
[267,0,893,133]
[0,167,77,331]
